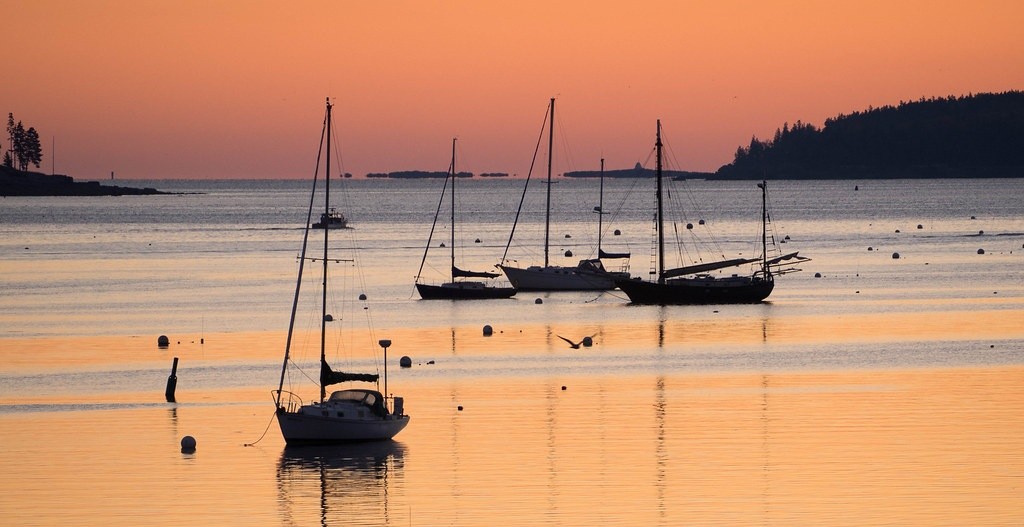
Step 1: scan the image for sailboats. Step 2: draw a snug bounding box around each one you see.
[416,137,520,302]
[612,118,778,305]
[273,94,412,441]
[483,96,633,292]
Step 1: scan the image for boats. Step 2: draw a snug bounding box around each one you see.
[311,207,351,229]
